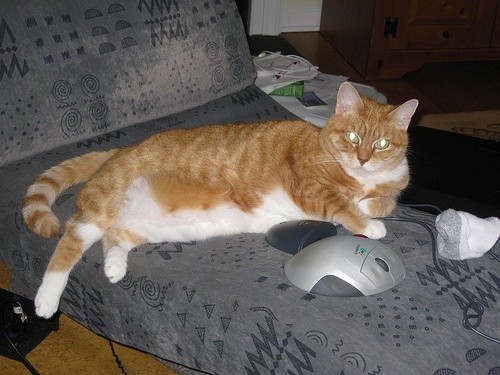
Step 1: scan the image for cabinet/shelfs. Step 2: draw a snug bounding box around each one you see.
[319,1,500,80]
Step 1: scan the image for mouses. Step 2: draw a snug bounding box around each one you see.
[285,235,408,297]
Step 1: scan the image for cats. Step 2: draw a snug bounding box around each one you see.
[21,81,420,318]
